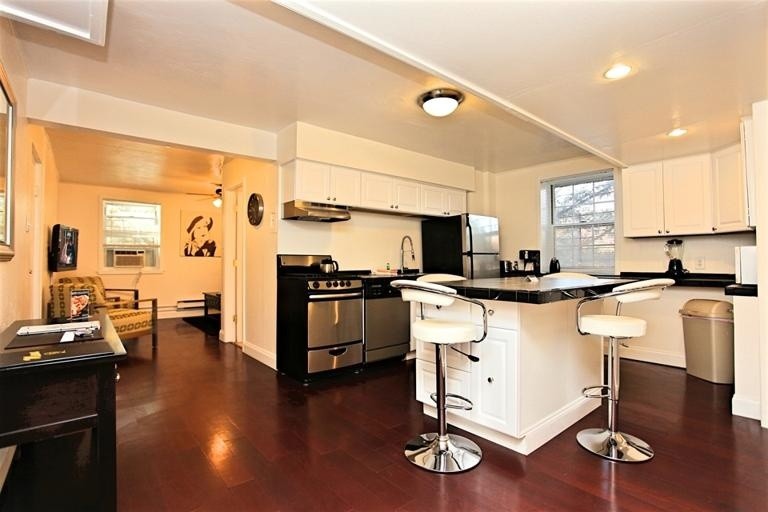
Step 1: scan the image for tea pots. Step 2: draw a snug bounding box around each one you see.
[320,260,339,276]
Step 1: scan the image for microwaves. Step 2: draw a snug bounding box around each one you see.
[109,249,145,268]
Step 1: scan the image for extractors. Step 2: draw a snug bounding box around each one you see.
[282,201,350,222]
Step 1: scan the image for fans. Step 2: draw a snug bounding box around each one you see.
[197,185,223,203]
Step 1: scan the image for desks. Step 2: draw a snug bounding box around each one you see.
[1,320,129,512]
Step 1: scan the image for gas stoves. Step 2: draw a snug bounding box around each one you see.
[279,272,363,281]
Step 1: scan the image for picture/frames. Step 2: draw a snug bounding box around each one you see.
[179,207,223,258]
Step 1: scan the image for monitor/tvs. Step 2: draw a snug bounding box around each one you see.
[51,224,79,273]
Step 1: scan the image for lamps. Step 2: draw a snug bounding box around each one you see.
[416,88,465,119]
[213,199,222,208]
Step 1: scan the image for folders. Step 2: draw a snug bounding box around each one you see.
[4,321,104,351]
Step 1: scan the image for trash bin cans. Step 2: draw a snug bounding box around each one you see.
[678,298,735,385]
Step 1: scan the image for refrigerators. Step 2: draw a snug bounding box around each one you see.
[422,214,499,280]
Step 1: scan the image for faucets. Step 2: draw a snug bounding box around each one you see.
[401,236,415,275]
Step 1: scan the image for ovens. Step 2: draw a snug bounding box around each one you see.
[278,282,363,348]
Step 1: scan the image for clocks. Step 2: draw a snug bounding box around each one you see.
[246,194,265,226]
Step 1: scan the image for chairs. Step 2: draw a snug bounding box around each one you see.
[391,278,487,475]
[45,276,161,348]
[574,278,673,464]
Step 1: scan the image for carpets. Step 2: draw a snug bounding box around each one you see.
[182,313,221,336]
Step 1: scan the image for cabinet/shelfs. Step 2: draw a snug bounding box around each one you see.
[362,170,421,215]
[281,160,362,208]
[622,152,715,237]
[739,117,756,226]
[715,143,753,233]
[421,181,467,217]
